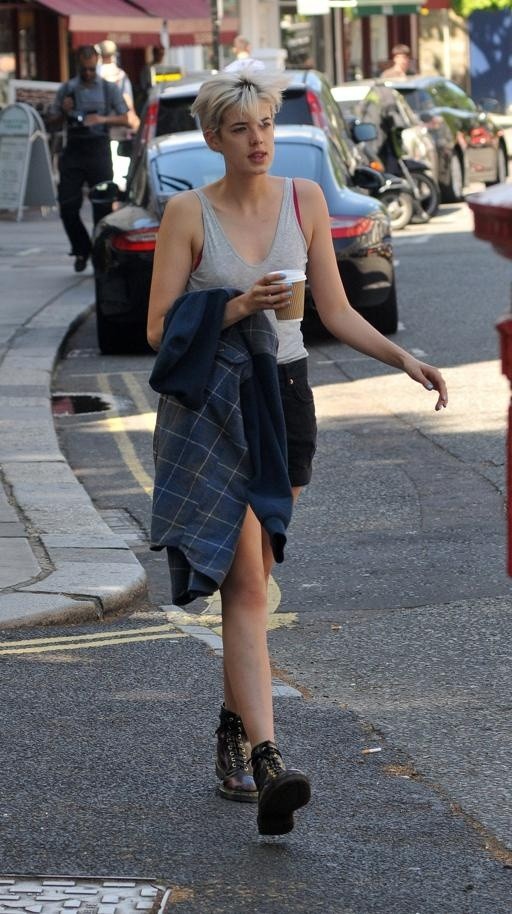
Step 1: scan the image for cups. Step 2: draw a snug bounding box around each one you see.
[269,268,306,324]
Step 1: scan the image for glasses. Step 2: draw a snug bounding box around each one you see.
[80,67,96,72]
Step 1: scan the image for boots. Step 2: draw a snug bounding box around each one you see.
[215,701,310,834]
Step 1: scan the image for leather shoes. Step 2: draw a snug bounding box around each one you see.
[75,242,91,272]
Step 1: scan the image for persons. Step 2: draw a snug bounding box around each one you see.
[50,43,131,273]
[219,32,268,73]
[379,44,419,77]
[95,38,142,192]
[144,63,451,838]
[140,44,167,97]
